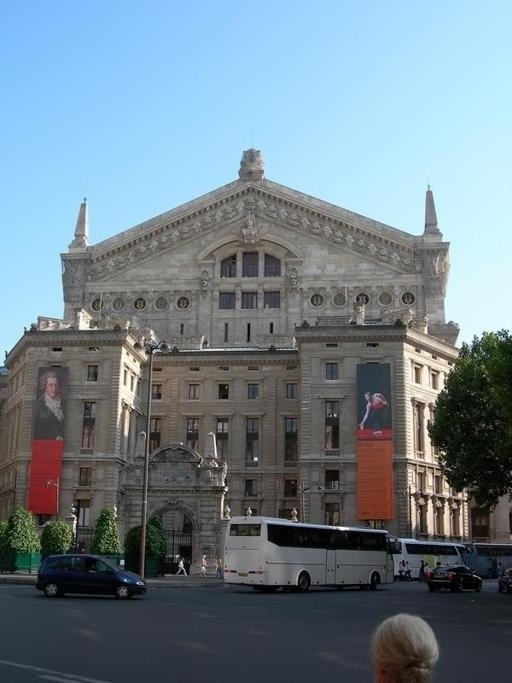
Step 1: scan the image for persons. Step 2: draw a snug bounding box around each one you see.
[419,560,425,583]
[35,372,64,440]
[372,614,439,683]
[358,392,388,431]
[487,558,493,579]
[89,564,98,573]
[200,555,208,577]
[398,562,403,582]
[405,561,411,582]
[424,563,428,583]
[176,558,188,576]
[216,557,223,578]
[496,562,503,579]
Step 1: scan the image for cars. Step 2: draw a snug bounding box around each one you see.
[424,564,483,592]
[497,568,512,593]
[36,553,147,599]
[164,554,186,572]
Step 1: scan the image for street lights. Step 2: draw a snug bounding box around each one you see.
[300,488,310,523]
[139,340,163,577]
[45,476,60,525]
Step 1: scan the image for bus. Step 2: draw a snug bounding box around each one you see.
[386,538,473,579]
[222,515,397,592]
[461,542,511,578]
